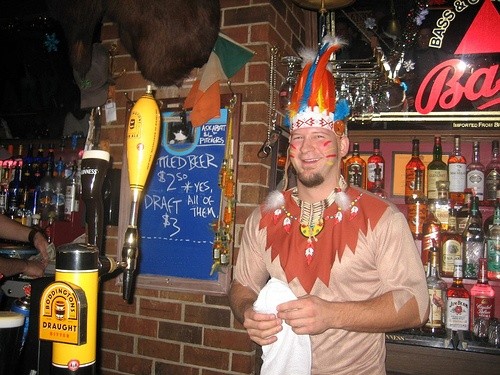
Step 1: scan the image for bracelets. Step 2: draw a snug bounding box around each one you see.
[29,228,38,243]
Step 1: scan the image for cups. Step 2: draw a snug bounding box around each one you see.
[0,311,25,375]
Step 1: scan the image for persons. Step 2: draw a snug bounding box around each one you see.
[0,214,49,277]
[228,36,430,375]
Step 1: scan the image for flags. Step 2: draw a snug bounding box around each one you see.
[184,35,254,128]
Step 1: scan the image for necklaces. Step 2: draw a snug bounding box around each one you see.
[274,192,364,264]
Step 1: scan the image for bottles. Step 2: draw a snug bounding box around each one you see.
[425,247,446,337]
[75,167,82,200]
[427,138,448,204]
[0,143,65,182]
[483,203,500,281]
[367,139,385,198]
[5,208,39,226]
[420,199,441,278]
[406,170,427,240]
[467,140,485,206]
[431,180,453,232]
[456,189,482,236]
[462,210,484,279]
[469,257,495,342]
[483,141,500,206]
[405,139,425,201]
[444,258,469,341]
[61,132,82,153]
[345,143,366,190]
[448,135,467,206]
[0,178,80,223]
[440,207,464,277]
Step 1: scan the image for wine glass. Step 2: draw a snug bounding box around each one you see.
[278,55,375,113]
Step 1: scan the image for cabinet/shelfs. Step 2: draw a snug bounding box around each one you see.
[0,137,88,247]
[346,112,500,354]
